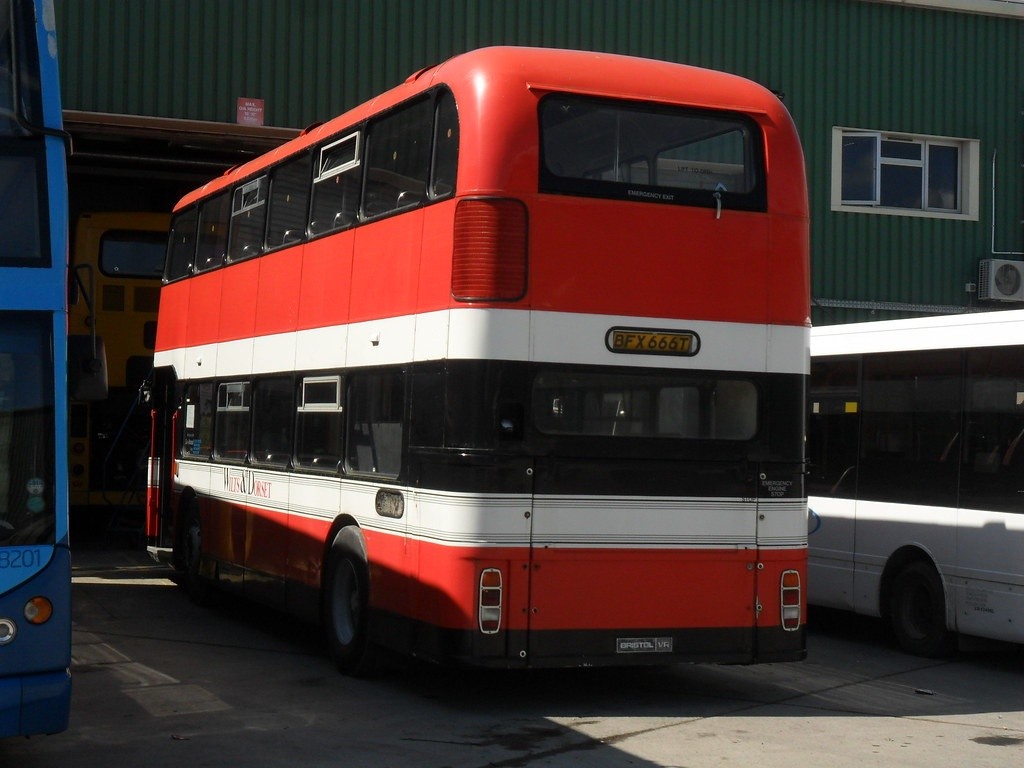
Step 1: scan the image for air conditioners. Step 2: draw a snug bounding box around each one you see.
[977,259,1024,301]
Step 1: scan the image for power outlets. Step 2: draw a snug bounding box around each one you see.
[965,283,977,293]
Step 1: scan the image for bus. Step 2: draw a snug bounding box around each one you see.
[72,209,248,516]
[807,308,1024,659]
[0,0,110,718]
[137,46,809,677]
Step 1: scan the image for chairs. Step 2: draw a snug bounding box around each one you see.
[811,411,1024,495]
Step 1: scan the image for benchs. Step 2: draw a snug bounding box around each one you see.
[188,159,746,476]
